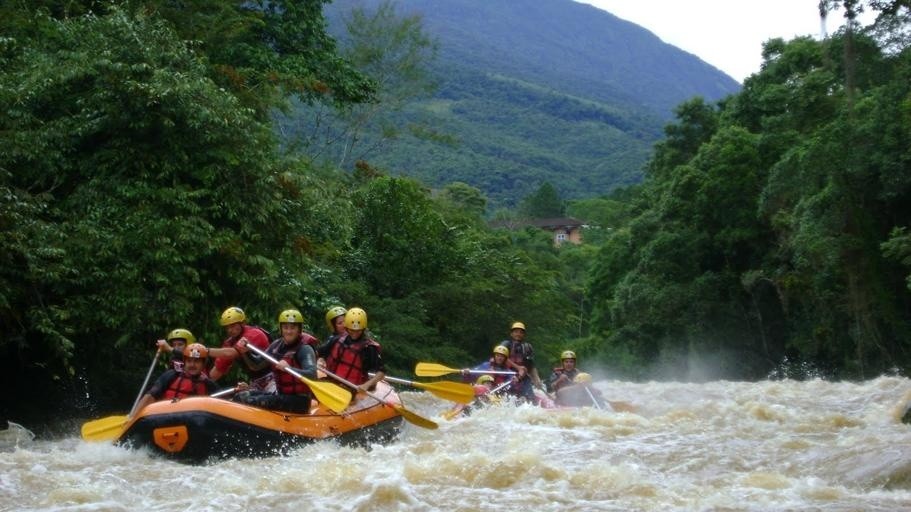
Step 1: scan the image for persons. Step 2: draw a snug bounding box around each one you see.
[123,306,387,425]
[461,321,601,406]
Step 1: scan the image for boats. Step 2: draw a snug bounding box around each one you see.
[113,376,403,465]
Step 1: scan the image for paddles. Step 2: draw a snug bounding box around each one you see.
[416,362,516,377]
[436,380,514,422]
[80,384,241,443]
[317,365,438,430]
[366,372,475,403]
[246,343,352,412]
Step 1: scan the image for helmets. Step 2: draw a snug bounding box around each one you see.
[344,307,368,330]
[279,310,303,335]
[561,350,577,370]
[492,345,509,360]
[182,342,209,358]
[220,306,246,326]
[325,306,348,334]
[475,375,494,386]
[167,328,196,347]
[509,321,526,331]
[573,372,592,385]
